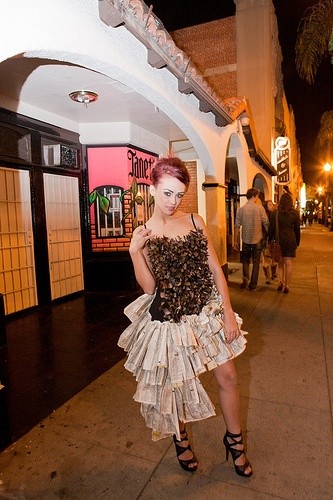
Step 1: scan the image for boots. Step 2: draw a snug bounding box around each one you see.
[262,265,271,284]
[271,264,278,280]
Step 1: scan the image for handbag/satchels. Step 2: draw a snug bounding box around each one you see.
[269,239,281,262]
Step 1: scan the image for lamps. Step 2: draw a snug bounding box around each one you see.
[69,91,99,110]
[237,112,251,126]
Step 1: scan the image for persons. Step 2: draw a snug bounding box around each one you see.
[299,210,318,226]
[232,188,271,289]
[268,194,302,294]
[127,156,255,477]
[260,199,280,284]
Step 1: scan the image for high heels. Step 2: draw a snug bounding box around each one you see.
[171,427,198,472]
[222,430,253,478]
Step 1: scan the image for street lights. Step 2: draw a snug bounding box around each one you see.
[324,162,331,226]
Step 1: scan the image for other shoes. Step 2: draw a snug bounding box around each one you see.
[240,278,256,289]
[277,283,289,293]
